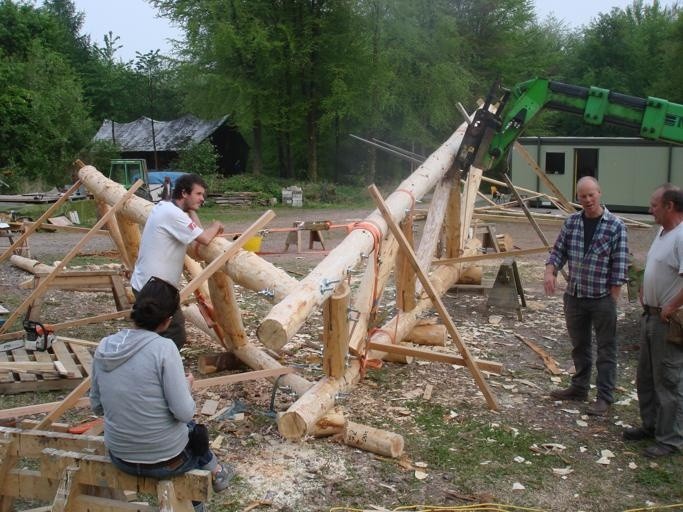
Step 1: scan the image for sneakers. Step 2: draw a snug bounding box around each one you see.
[645,443,673,457]
[212,465,236,493]
[551,388,588,400]
[587,397,612,415]
[623,427,652,441]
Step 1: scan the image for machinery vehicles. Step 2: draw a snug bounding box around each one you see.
[456,73,683,177]
[97,155,152,230]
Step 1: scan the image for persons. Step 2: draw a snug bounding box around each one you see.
[88,276,237,512]
[127,173,223,357]
[621,181,682,457]
[542,176,628,415]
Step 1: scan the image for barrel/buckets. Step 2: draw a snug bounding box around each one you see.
[240,235,262,252]
[287,185,301,192]
[292,190,303,207]
[282,187,293,205]
